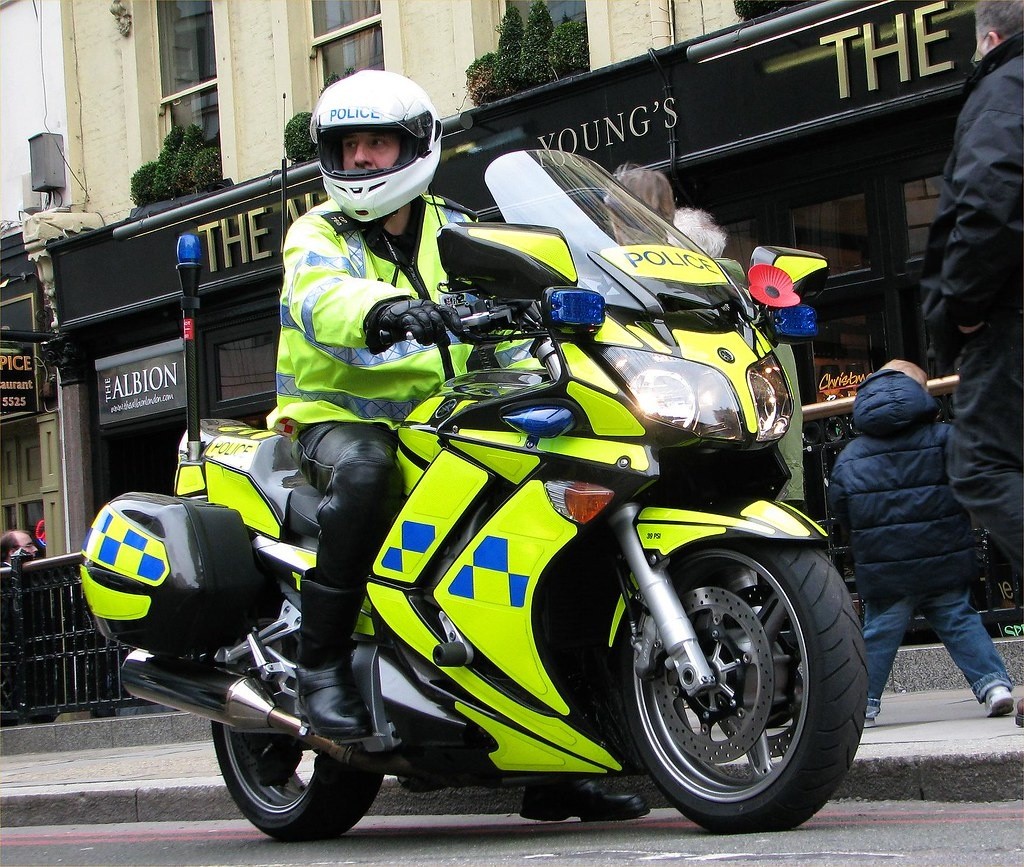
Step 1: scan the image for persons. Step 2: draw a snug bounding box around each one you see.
[0,528,39,568]
[920,0,1024,727]
[265,68,537,741]
[828,358,1015,729]
[602,162,729,258]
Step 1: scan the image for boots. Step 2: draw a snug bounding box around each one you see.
[294,566,375,740]
[520,778,650,821]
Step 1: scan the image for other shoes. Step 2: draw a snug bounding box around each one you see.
[1016,698,1024,727]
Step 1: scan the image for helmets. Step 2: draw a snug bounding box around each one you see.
[317,71,443,222]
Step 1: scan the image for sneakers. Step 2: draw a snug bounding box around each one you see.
[984,686,1014,717]
[863,718,876,727]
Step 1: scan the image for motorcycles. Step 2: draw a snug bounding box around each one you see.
[76,148,871,845]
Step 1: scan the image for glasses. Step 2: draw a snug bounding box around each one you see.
[18,543,34,550]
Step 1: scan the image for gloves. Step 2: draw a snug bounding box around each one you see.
[376,299,463,346]
